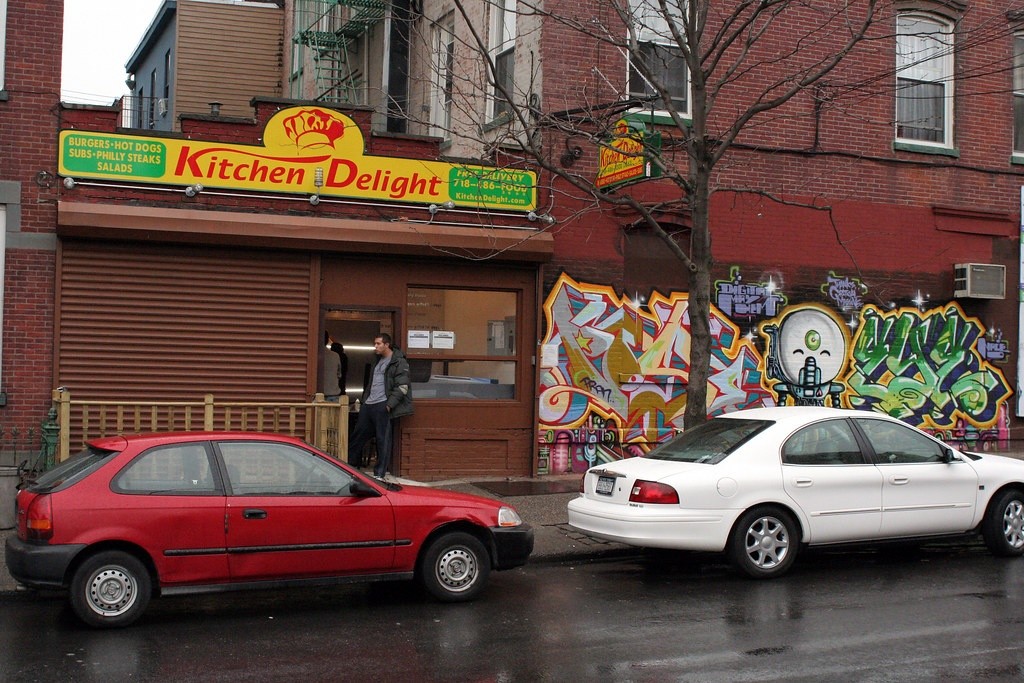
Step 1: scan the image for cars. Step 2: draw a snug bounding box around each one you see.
[565,406,1024,582]
[4,430,536,631]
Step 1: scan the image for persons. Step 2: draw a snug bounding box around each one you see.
[324,329,348,398]
[348,333,415,477]
